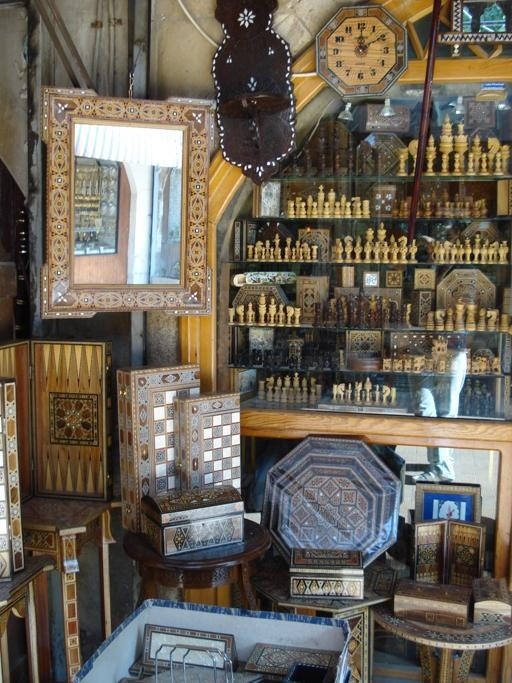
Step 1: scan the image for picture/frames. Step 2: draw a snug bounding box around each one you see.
[414,482,483,524]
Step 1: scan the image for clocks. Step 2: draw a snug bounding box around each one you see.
[312,2,409,102]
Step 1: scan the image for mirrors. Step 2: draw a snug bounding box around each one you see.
[35,83,214,320]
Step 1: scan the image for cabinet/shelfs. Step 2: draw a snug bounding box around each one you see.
[171,1,510,683]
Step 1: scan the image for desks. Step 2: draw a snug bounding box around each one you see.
[124,516,510,681]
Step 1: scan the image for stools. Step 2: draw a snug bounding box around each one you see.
[2,496,125,681]
